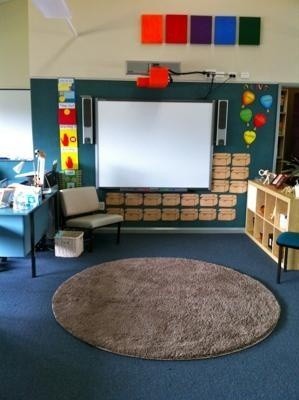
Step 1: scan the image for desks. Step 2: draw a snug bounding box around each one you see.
[0,188,58,279]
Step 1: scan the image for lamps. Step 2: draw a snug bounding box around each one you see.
[14,158,36,187]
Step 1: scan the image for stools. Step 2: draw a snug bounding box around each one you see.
[275,231,299,284]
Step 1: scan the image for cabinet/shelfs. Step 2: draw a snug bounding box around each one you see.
[276,88,288,171]
[243,179,299,271]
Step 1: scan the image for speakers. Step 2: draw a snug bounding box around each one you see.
[80,95,94,145]
[215,99,229,146]
[126,60,182,76]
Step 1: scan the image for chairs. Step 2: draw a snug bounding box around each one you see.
[58,185,124,252]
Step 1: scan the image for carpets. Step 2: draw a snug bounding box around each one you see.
[50,255,280,361]
